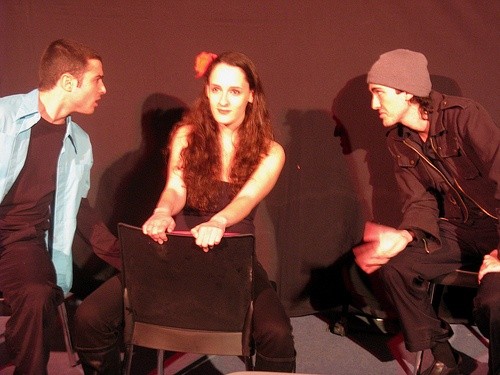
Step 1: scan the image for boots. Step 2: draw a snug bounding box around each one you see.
[74,336,121,375]
[255,349,296,373]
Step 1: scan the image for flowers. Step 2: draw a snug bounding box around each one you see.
[193,52,218,80]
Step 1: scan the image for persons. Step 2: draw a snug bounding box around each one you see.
[353,49,500,375]
[0,39,124,375]
[73,50,297,375]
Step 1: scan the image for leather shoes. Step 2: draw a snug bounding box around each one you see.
[421,356,462,375]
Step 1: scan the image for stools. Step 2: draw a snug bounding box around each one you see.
[0,292,78,369]
[412,268,500,375]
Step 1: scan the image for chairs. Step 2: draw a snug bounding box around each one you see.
[116,223,258,375]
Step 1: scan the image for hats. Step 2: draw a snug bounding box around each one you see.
[367,49,431,96]
